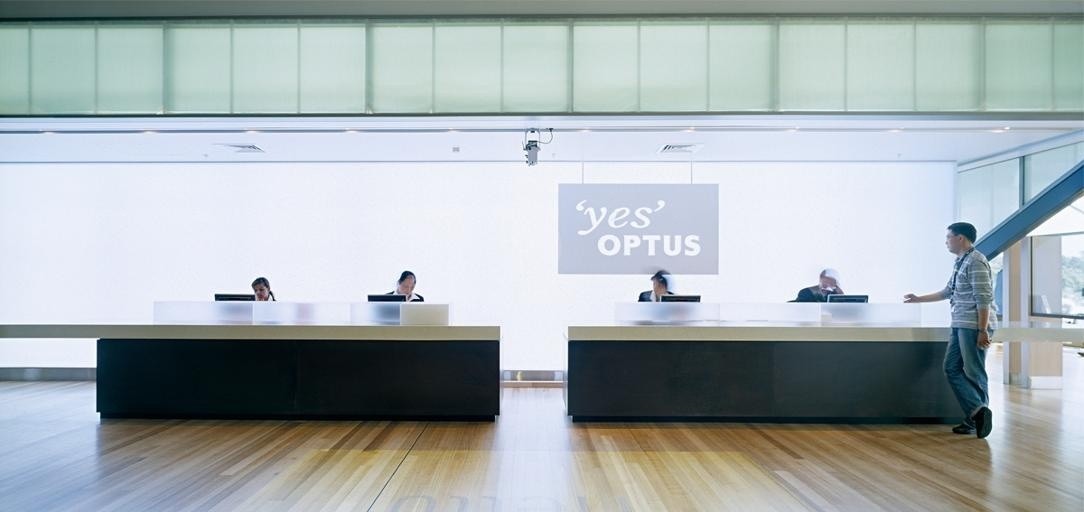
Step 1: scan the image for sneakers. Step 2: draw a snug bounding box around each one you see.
[952,421,975,438]
[973,407,992,438]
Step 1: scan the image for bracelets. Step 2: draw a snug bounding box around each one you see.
[977,328,987,333]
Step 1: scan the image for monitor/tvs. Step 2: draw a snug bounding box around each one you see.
[367,295,406,302]
[214,294,255,300]
[827,294,868,302]
[660,294,701,301]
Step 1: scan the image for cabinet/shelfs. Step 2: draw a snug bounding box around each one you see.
[97,335,501,421]
[563,335,976,429]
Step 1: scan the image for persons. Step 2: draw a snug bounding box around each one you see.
[250,276,276,301]
[903,222,995,440]
[384,270,424,303]
[637,269,676,301]
[787,267,845,302]
